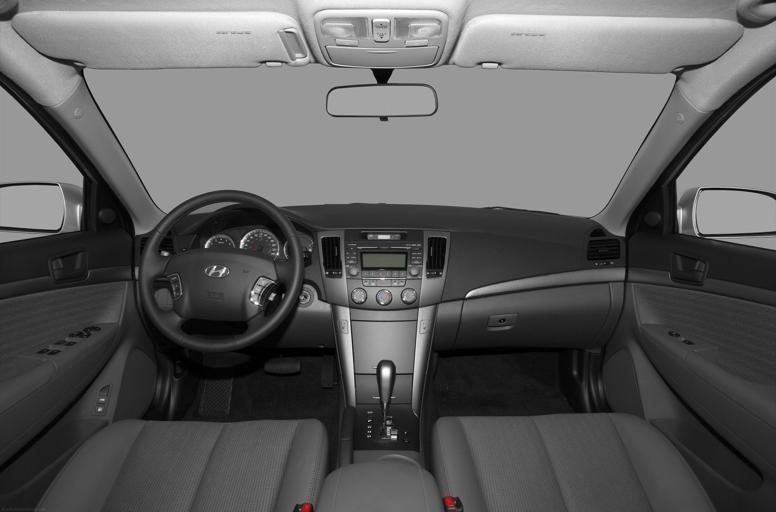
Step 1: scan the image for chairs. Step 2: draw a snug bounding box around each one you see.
[34,412,328,512]
[431,412,717,512]
